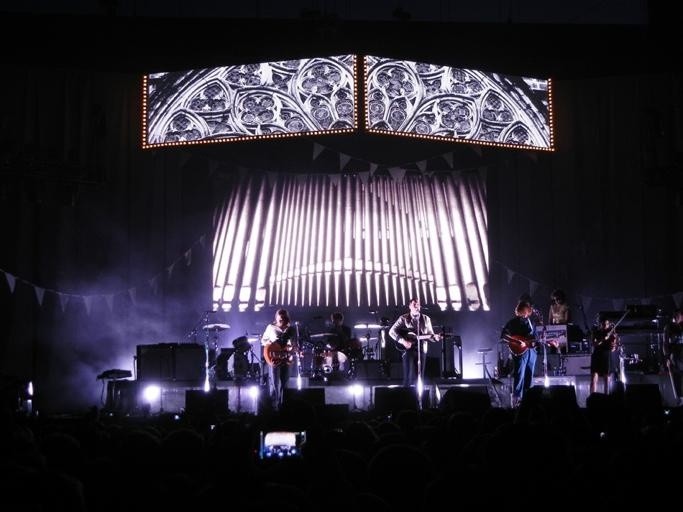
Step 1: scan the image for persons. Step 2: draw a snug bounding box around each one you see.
[501,300,541,406]
[591,316,616,392]
[549,287,571,326]
[662,308,683,405]
[1,387,683,512]
[388,298,441,388]
[325,312,351,351]
[261,308,304,407]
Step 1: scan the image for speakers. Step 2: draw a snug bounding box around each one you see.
[281,387,326,413]
[136,342,174,382]
[184,389,228,415]
[532,353,560,377]
[624,382,661,408]
[438,386,491,412]
[174,342,207,386]
[374,386,417,411]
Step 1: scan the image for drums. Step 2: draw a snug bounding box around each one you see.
[322,348,351,379]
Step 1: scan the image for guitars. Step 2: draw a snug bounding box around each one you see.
[395,330,454,352]
[262,341,322,370]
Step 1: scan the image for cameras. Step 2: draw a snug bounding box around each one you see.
[259,429,307,462]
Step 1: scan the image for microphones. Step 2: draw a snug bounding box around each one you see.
[416,308,426,314]
[295,321,300,338]
[532,308,544,313]
